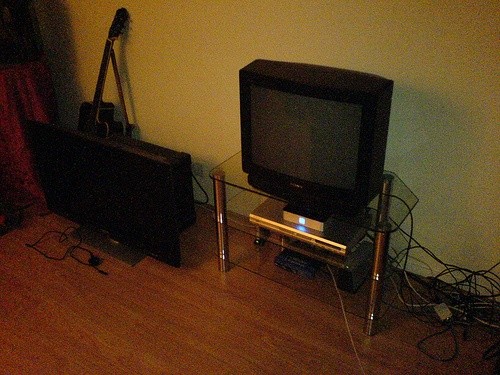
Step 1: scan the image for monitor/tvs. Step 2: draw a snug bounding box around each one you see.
[239,59,393,209]
[24,118,181,268]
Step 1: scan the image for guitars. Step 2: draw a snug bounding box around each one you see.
[78,7,135,140]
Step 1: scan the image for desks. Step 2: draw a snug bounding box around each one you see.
[209,151,420,337]
